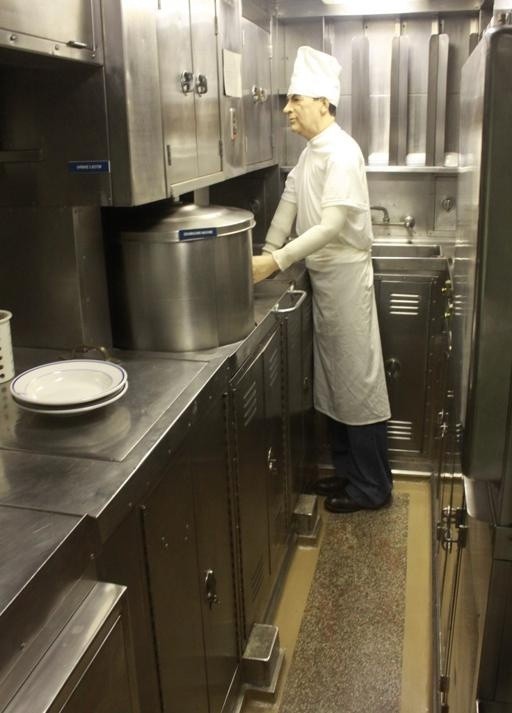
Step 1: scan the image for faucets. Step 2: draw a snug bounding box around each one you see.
[370,205,390,223]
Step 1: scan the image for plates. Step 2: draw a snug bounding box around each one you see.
[7,378,129,416]
[13,407,131,454]
[10,356,128,408]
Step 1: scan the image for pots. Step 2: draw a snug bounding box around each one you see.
[100,194,257,351]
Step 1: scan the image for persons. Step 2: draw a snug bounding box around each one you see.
[250,43,393,515]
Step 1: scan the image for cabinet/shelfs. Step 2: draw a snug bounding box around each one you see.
[154,0,223,199]
[0,0,105,71]
[241,0,279,174]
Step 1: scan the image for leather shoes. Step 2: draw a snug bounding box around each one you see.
[313,473,352,496]
[324,489,391,513]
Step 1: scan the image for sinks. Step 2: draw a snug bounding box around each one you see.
[370,244,449,273]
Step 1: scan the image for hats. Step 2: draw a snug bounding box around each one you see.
[286,44,342,107]
